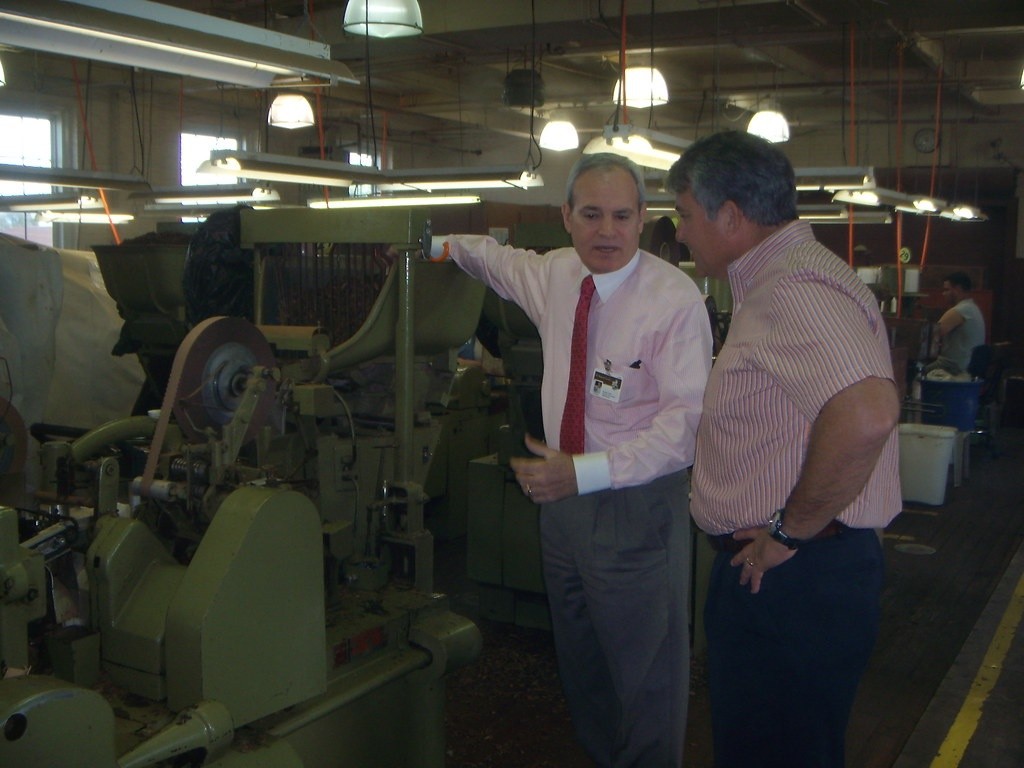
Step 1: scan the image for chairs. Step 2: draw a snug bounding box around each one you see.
[980,340,1012,458]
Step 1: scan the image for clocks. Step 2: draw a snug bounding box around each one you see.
[913,128,935,153]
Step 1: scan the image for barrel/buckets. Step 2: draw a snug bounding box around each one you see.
[897,423,958,506]
[916,377,986,431]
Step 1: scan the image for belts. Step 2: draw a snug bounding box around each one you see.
[706,520,849,551]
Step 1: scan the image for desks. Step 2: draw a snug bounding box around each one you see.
[954,431,970,487]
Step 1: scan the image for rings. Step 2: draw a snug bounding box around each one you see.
[746,558,755,567]
[526,484,533,496]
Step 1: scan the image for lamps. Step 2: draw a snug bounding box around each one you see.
[602,123,680,170]
[0,1,360,90]
[268,93,315,129]
[612,67,668,108]
[646,166,988,225]
[342,0,424,39]
[747,111,789,143]
[0,149,544,224]
[539,104,583,149]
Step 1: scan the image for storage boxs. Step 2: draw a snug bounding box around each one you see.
[899,423,958,505]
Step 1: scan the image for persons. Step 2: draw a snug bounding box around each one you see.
[924,270,986,373]
[664,128,904,768]
[384,151,714,768]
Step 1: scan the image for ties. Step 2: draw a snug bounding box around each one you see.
[559,274,596,455]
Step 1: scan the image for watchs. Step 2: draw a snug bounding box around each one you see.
[768,507,813,551]
